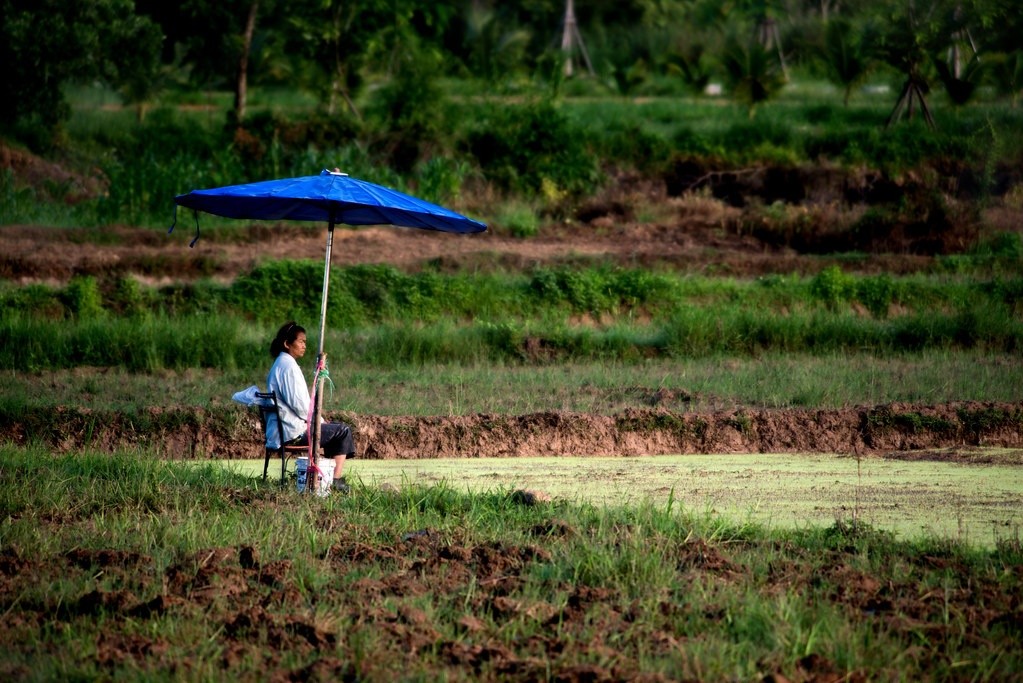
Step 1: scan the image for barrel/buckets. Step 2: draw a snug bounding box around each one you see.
[294,457,337,497]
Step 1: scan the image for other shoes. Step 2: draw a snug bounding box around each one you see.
[331,477,350,494]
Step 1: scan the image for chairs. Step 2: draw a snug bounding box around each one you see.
[255,391,327,488]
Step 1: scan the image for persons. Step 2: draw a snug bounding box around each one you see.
[267,322,356,494]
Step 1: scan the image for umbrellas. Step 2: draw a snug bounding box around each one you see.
[176,167,487,459]
